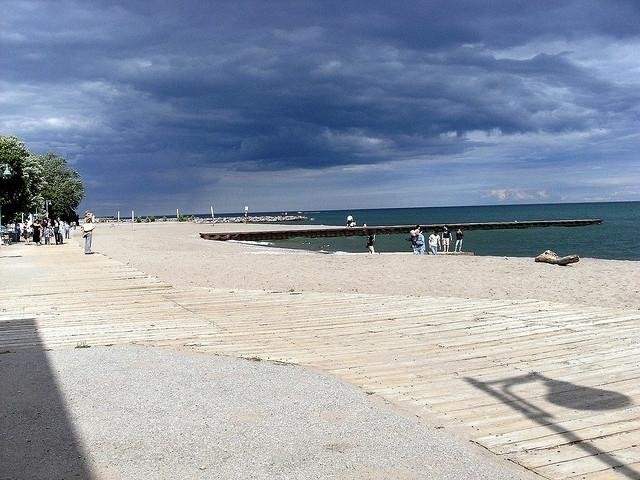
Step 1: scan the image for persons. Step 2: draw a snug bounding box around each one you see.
[366,234,376,254]
[346,214,354,227]
[454,226,464,252]
[82,219,93,238]
[410,224,421,253]
[15,216,77,246]
[416,229,425,255]
[428,228,442,255]
[81,218,96,254]
[441,225,453,252]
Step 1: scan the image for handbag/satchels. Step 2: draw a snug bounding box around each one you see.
[84,223,93,231]
